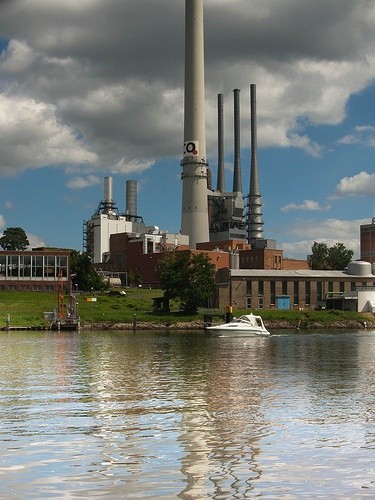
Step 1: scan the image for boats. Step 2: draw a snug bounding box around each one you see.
[204,311,269,336]
